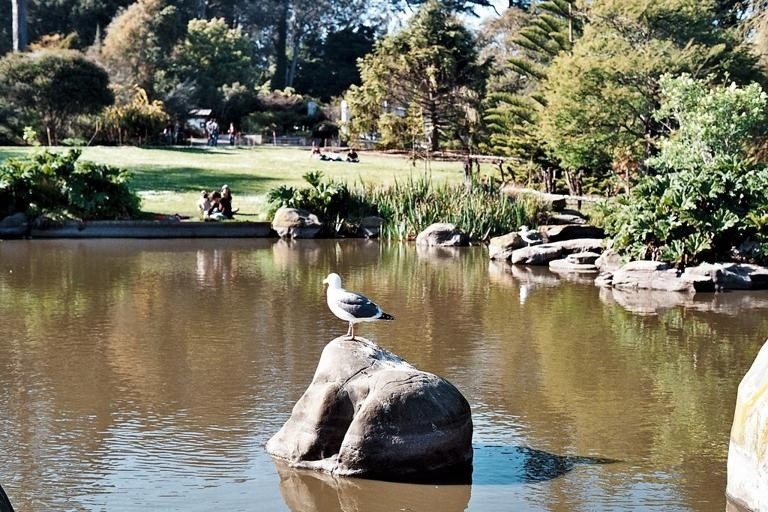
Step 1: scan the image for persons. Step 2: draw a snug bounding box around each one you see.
[206,117,220,147]
[163,120,184,144]
[228,123,237,146]
[309,140,359,162]
[199,185,239,222]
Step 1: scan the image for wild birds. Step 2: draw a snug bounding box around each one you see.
[323,273,396,341]
[519,225,546,248]
